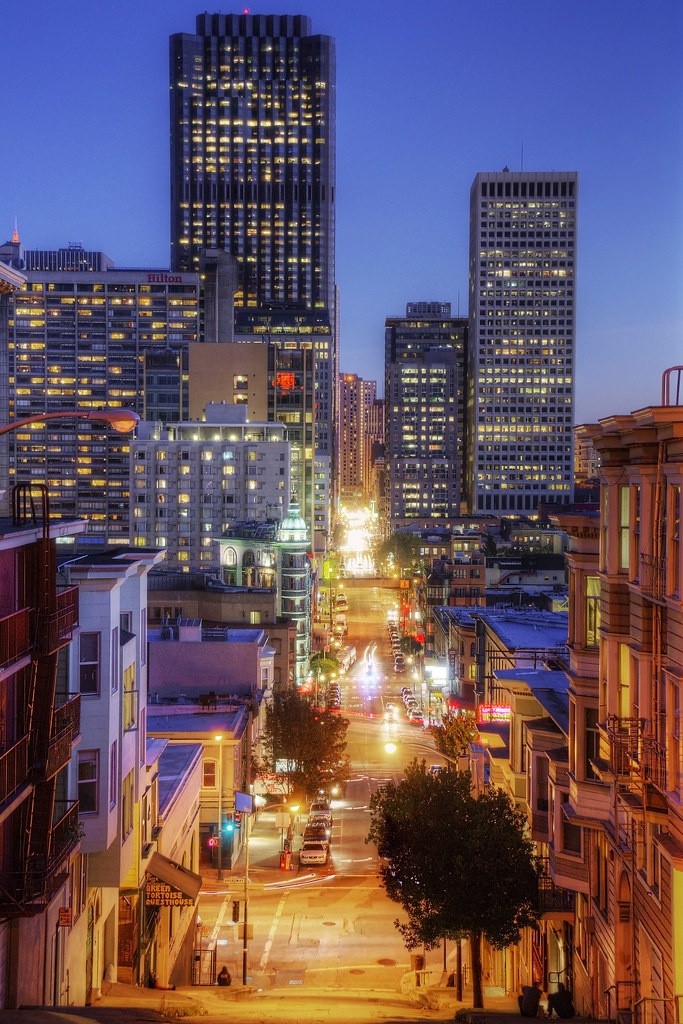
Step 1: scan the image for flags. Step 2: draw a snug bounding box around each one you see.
[234,791,253,815]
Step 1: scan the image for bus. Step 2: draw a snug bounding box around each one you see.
[334,644,357,673]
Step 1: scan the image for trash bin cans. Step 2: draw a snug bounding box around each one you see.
[281,852,292,870]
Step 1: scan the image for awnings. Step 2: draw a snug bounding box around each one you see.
[146,851,203,906]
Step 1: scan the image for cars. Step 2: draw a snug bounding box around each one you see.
[299,790,332,867]
[333,614,350,638]
[401,680,424,727]
[335,591,350,613]
[388,616,405,671]
[328,679,343,711]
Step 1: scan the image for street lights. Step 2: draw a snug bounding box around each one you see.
[385,740,464,1001]
[329,568,340,632]
[242,796,300,986]
[0,408,142,434]
[211,729,226,879]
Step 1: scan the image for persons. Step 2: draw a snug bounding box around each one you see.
[217,967,232,987]
